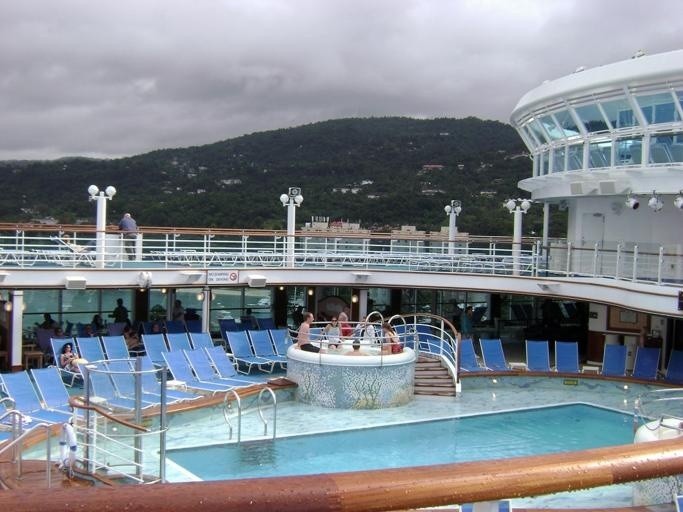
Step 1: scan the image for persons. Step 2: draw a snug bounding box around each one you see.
[458,306,481,360]
[171,299,186,320]
[245,308,257,330]
[62,234,96,253]
[117,212,139,260]
[33,312,160,374]
[290,302,402,356]
[107,298,127,323]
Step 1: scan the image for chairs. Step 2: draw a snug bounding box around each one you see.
[0,316,298,440]
[396,325,452,371]
[526,340,550,371]
[521,254,547,274]
[310,328,323,343]
[555,341,579,373]
[452,338,484,372]
[479,338,508,371]
[632,346,661,379]
[665,349,683,381]
[151,250,285,267]
[601,344,627,377]
[295,251,512,274]
[0,236,96,266]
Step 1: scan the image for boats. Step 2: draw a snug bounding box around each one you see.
[245,286,301,314]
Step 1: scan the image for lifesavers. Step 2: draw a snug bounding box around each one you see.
[58,421,77,468]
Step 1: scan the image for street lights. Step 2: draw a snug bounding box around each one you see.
[278,193,304,267]
[87,183,118,266]
[444,205,462,254]
[501,197,533,276]
